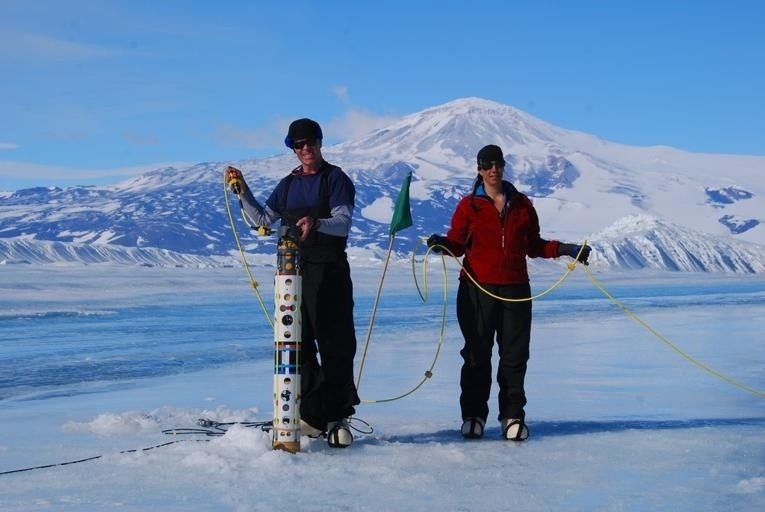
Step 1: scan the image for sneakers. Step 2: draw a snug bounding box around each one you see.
[461,416,486,438]
[326,417,353,447]
[501,417,529,441]
[298,418,322,438]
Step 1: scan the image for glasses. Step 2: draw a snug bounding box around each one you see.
[293,138,317,149]
[478,158,506,170]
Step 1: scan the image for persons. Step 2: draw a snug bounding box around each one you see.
[427,144,587,438]
[226,119,360,447]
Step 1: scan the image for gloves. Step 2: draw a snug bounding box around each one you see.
[558,243,592,265]
[427,234,448,253]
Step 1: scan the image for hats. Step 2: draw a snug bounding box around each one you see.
[284,119,322,139]
